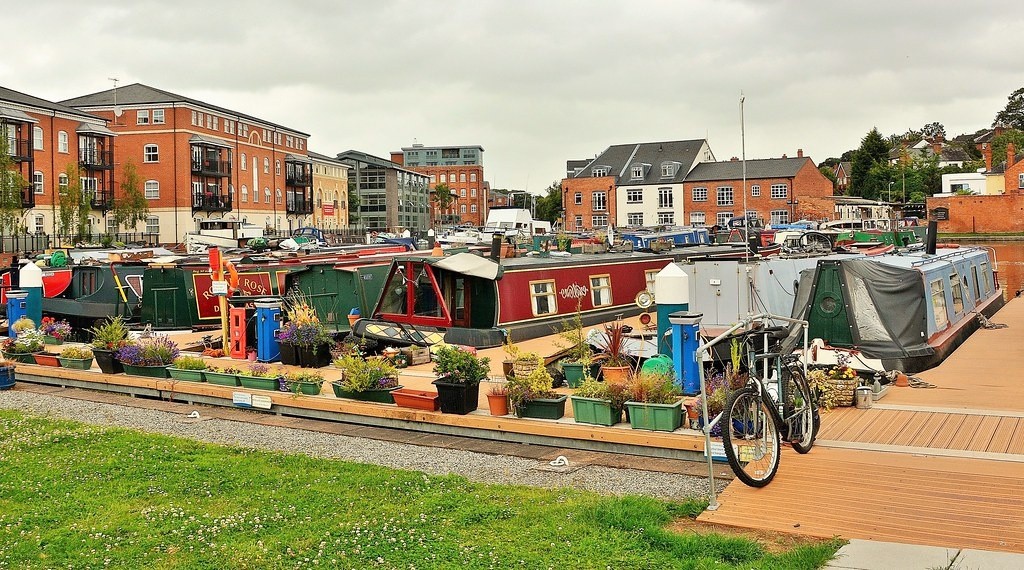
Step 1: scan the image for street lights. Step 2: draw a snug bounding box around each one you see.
[888,182,894,202]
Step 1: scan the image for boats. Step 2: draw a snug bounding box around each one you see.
[790,220,1005,385]
[0,205,939,363]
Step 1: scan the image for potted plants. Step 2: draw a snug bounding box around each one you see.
[0,316,876,435]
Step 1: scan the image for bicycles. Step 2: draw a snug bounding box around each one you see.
[722,326,816,487]
